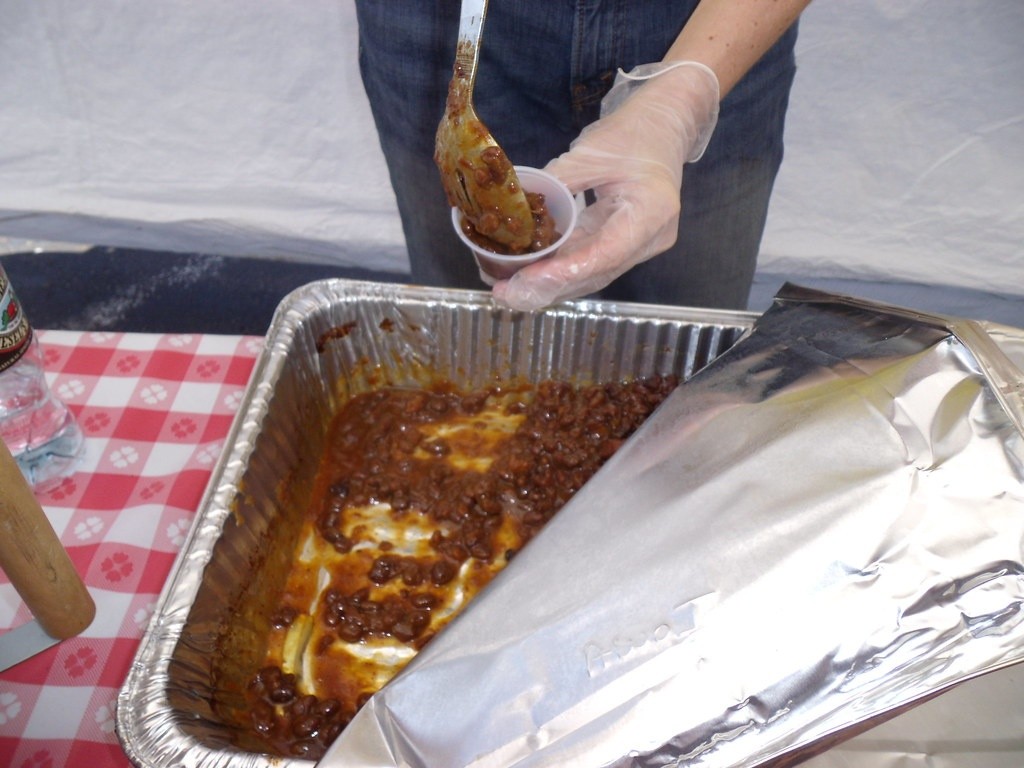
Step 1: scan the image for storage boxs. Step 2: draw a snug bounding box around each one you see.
[129,266,1024,768]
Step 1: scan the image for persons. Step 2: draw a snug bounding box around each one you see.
[355,0,810,312]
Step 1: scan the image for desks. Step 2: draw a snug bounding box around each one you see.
[0,321,261,768]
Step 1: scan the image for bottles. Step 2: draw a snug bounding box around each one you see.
[1,263,85,495]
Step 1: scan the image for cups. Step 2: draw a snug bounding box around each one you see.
[453,166,579,287]
